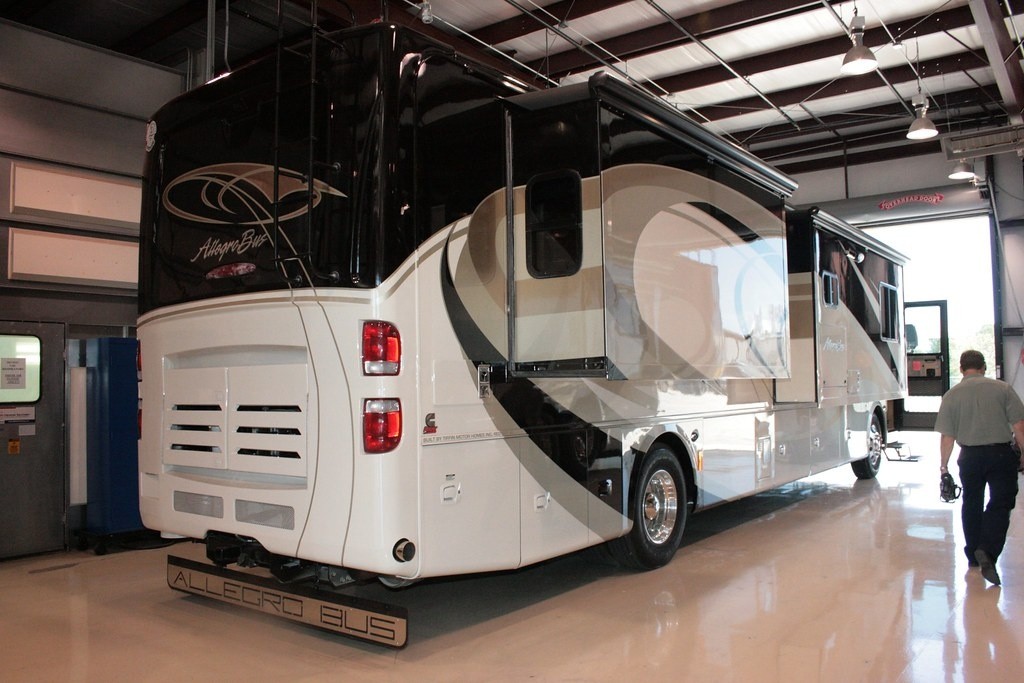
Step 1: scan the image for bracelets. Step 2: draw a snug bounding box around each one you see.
[940,465,948,470]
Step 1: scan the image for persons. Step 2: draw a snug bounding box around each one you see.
[933,351,1024,585]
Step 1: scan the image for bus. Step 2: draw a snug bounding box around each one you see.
[133,20,950,650]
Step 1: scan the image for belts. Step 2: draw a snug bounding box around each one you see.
[990,442,1009,447]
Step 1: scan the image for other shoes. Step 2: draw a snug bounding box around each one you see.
[975,549,1001,585]
[965,545,976,564]
